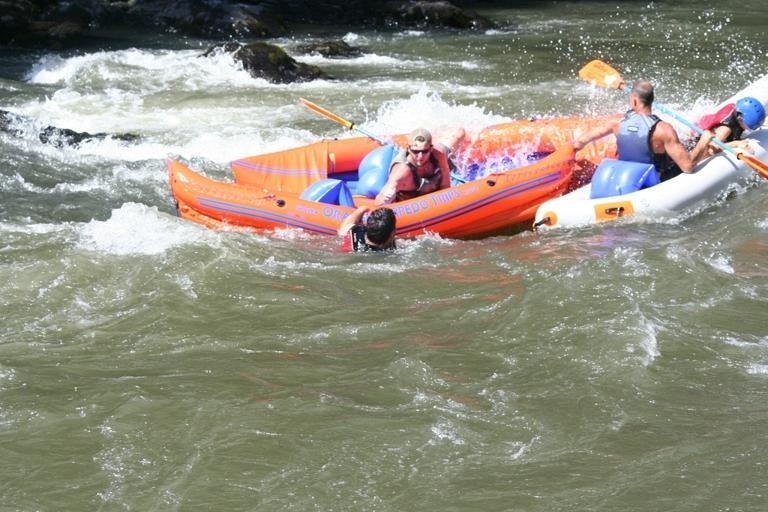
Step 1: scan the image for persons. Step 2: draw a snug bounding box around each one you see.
[571,80,714,182]
[330,205,396,253]
[373,128,451,209]
[689,96,765,156]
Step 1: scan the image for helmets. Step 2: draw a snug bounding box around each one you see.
[735,96,767,131]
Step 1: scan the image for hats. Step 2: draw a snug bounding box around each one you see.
[407,128,433,147]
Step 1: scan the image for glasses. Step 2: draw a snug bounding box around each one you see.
[409,145,430,155]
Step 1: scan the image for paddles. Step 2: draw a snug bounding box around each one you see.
[578,59,768,179]
[300,97,388,146]
